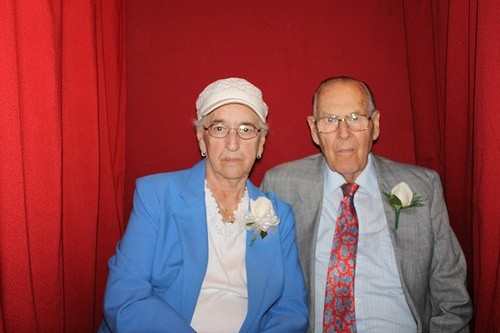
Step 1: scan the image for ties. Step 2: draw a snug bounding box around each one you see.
[323,183,360,332]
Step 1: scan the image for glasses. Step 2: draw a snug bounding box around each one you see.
[313,113,371,133]
[204,124,263,139]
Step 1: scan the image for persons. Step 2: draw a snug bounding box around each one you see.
[99,77,309,333]
[258,75,471,332]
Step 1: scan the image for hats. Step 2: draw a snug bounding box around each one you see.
[197,77,269,125]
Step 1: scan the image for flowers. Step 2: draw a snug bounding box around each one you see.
[382,181,431,231]
[244,196,282,246]
